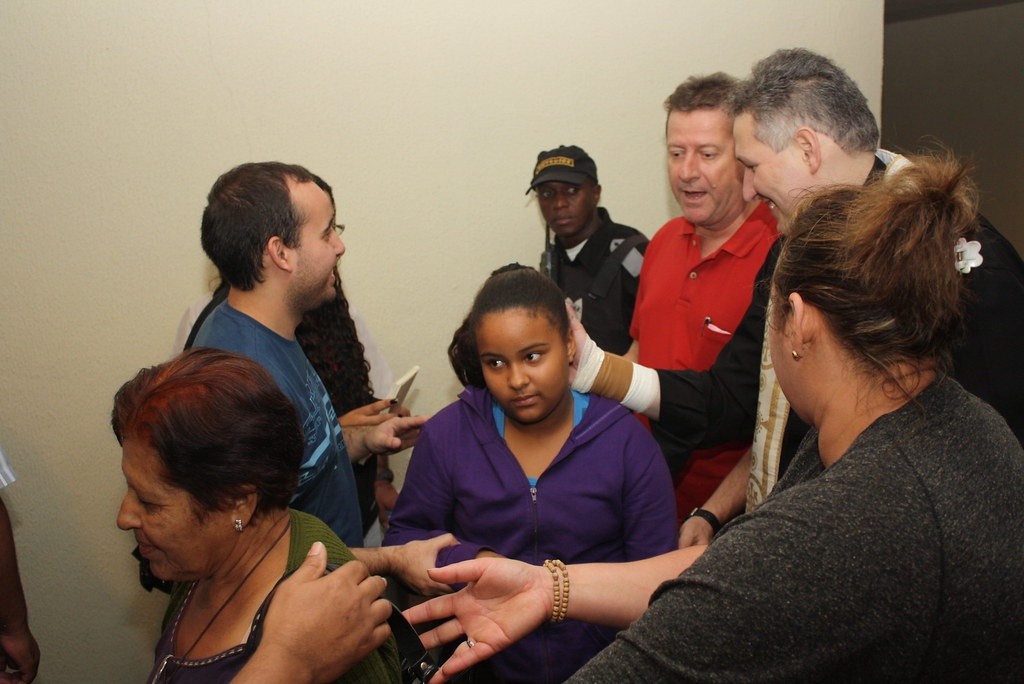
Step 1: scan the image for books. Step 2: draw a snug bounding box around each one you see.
[381,366,419,414]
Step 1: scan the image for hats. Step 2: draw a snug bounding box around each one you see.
[526,145,598,196]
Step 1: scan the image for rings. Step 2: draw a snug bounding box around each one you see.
[466,640,473,647]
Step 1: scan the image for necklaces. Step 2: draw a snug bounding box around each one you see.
[165,520,291,684]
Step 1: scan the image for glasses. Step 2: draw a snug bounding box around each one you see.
[334,224,346,236]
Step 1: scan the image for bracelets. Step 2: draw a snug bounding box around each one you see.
[590,351,633,401]
[545,559,569,623]
[620,362,657,413]
[683,508,720,535]
[376,469,394,483]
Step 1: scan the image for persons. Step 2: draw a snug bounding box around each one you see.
[562,48,1023,554]
[380,263,681,684]
[0,449,41,684]
[233,540,393,684]
[114,346,415,684]
[525,146,652,355]
[177,160,429,544]
[403,154,1024,684]
[626,72,782,549]
